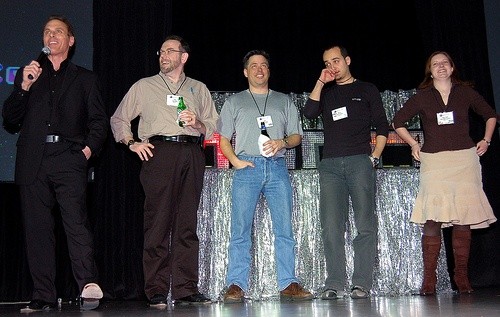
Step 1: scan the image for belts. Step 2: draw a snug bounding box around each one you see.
[149,135,201,145]
[45,134,65,144]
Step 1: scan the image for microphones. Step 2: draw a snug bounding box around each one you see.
[28,46,51,79]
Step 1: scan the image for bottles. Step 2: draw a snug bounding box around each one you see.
[177,97,187,127]
[259,121,275,157]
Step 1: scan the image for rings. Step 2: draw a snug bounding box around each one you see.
[190,118,192,121]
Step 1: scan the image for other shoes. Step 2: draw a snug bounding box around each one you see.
[147,294,168,308]
[280,282,314,300]
[321,288,338,300]
[350,284,370,299]
[224,285,246,303]
[172,291,213,305]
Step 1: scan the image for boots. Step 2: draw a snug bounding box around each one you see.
[451,237,476,293]
[419,235,441,297]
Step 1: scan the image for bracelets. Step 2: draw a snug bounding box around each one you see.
[318,79,326,84]
[282,139,288,146]
[483,138,490,146]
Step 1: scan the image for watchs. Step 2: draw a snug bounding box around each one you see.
[127,140,137,149]
[370,155,379,165]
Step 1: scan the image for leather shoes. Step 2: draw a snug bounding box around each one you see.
[79,281,104,310]
[19,299,58,314]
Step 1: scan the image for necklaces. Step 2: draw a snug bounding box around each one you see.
[336,76,352,83]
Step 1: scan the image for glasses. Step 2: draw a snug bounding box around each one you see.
[156,48,184,56]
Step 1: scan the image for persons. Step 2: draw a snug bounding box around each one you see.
[302,41,389,299]
[110,35,220,306]
[391,51,497,296]
[0,16,107,312]
[216,49,312,303]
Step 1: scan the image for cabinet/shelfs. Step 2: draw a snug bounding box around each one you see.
[166,89,454,304]
